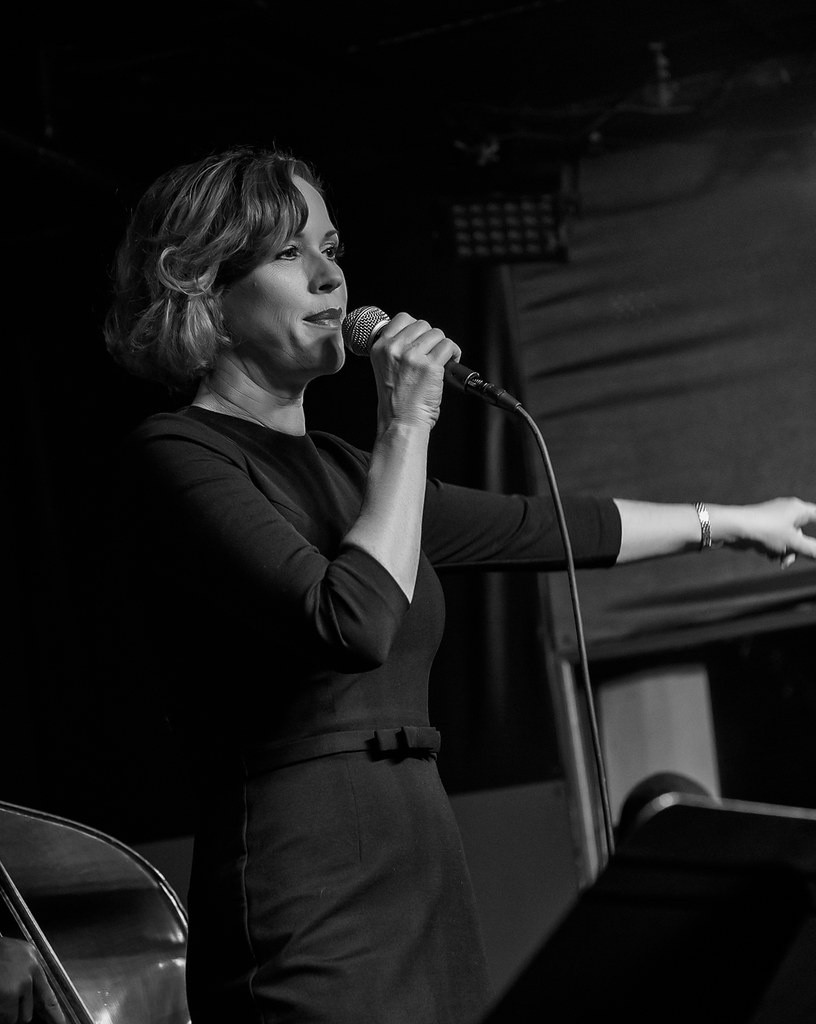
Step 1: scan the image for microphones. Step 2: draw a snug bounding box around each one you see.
[343,305,523,415]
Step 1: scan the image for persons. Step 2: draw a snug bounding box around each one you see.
[101,145,815,1024]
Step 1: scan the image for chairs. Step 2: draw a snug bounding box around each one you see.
[0,800,188,1022]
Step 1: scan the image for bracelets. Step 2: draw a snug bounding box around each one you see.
[695,500,712,554]
